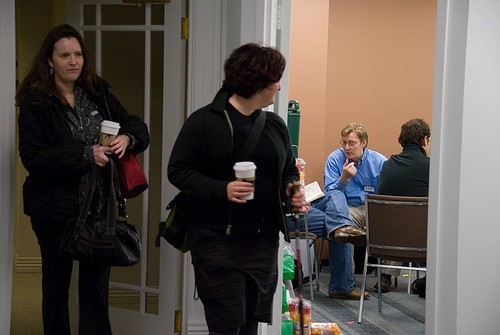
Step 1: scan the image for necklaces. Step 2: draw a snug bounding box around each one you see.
[73,99,83,131]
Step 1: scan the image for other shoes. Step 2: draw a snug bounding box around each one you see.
[334,225,367,245]
[329,287,370,299]
[411,276,426,294]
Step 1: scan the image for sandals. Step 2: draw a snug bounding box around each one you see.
[375,274,389,292]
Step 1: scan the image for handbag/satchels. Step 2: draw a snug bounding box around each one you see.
[65,156,140,268]
[98,85,148,199]
[159,190,189,254]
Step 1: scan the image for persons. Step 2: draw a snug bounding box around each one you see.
[285,190,371,299]
[376,118,431,294]
[169,43,311,335]
[323,123,403,291]
[14,23,151,335]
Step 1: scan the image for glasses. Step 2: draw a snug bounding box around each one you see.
[339,138,362,146]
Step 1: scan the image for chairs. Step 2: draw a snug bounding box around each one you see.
[286,212,320,301]
[358,193,429,324]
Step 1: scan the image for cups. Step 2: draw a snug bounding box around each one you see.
[233,160,257,200]
[98,120,121,155]
